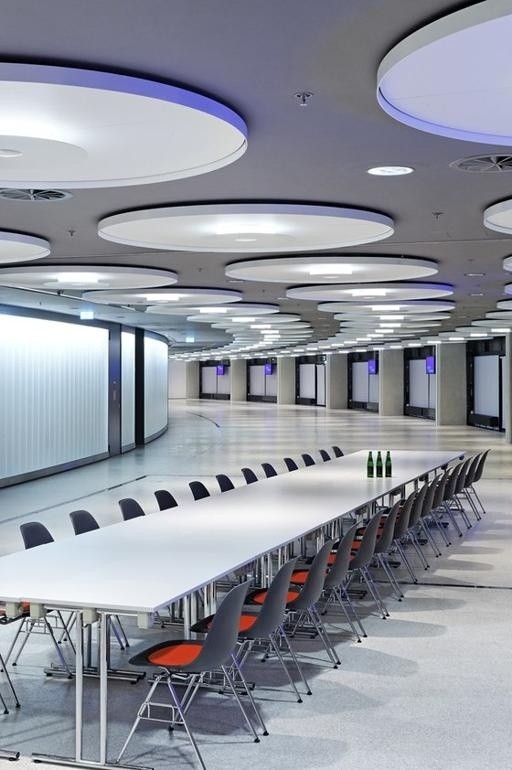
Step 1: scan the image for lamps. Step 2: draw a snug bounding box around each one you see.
[1,62,250,195]
[376,1,511,150]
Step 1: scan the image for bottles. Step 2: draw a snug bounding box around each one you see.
[367,451,374,477]
[385,451,391,477]
[376,451,382,477]
